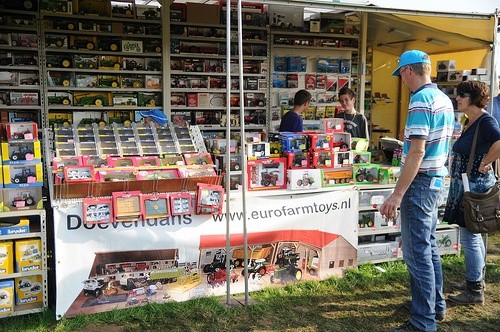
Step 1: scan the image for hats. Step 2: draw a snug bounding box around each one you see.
[391,50,431,76]
[139,108,168,126]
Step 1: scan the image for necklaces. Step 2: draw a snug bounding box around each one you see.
[343,111,357,129]
[464,109,482,127]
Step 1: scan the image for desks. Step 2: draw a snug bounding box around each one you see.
[53,184,358,320]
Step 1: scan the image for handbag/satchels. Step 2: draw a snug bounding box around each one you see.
[462,180,500,232]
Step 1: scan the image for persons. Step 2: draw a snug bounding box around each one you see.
[336,88,368,141]
[380,50,455,332]
[447,80,500,305]
[279,89,312,132]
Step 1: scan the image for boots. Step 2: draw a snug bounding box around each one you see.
[451,280,469,291]
[448,280,485,306]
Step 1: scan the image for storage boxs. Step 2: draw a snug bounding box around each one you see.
[0,0,493,315]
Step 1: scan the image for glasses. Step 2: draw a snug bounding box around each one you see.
[457,92,470,98]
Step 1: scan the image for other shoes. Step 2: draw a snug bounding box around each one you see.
[390,320,425,332]
[404,298,446,320]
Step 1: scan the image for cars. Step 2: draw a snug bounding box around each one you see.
[0,0,404,308]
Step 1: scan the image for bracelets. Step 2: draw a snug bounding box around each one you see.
[481,161,491,166]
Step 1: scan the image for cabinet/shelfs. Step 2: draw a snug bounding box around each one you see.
[41,10,169,125]
[169,19,271,129]
[0,9,47,181]
[358,187,464,265]
[0,207,49,321]
[270,30,360,132]
